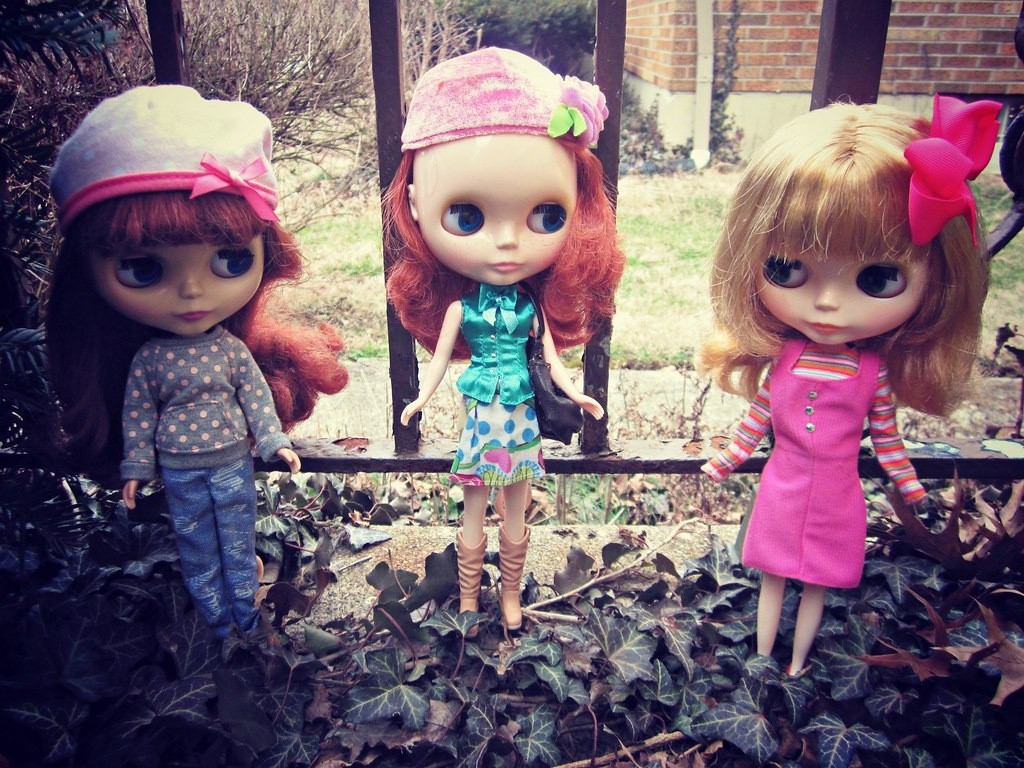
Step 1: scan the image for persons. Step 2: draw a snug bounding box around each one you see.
[383,44,628,638]
[35,82,348,672]
[700,92,1004,679]
